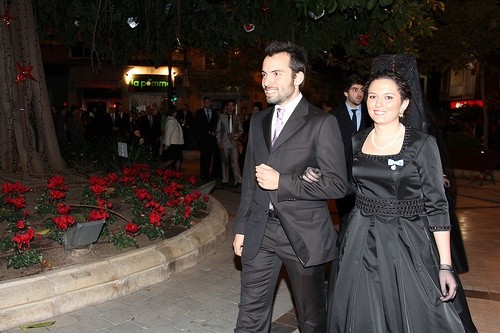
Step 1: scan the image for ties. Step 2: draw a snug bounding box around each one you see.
[271,107,286,147]
[206,110,210,121]
[112,112,113,118]
[150,115,151,124]
[120,111,121,118]
[350,109,357,134]
[228,114,233,133]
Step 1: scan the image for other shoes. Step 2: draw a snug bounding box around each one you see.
[200,175,211,183]
[235,178,243,184]
[222,175,229,184]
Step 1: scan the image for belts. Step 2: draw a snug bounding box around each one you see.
[268,209,278,219]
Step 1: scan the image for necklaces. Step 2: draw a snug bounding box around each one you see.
[370,123,404,150]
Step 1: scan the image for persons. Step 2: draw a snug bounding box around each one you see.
[230,38,348,333]
[302,56,479,332]
[324,75,375,230]
[48,96,450,193]
